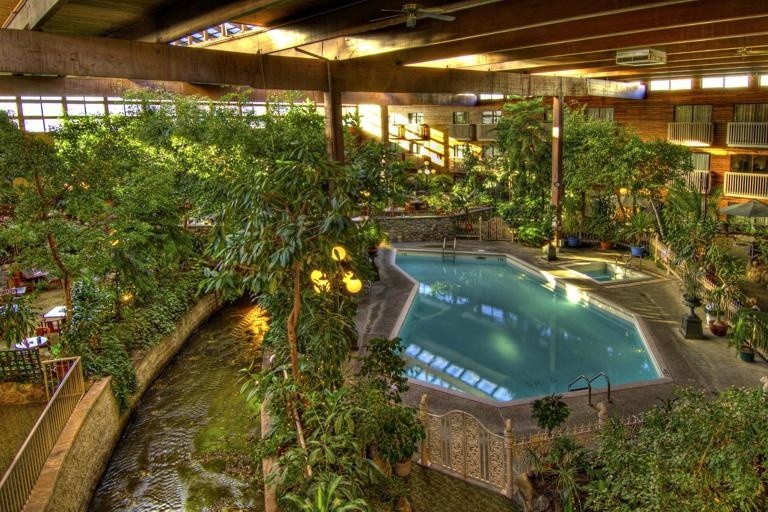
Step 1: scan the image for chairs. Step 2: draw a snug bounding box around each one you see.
[30,327,50,337]
[41,317,66,336]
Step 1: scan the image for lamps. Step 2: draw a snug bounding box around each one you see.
[310,240,363,314]
[724,307,768,363]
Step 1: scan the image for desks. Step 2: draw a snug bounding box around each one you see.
[44,305,76,320]
[15,336,49,349]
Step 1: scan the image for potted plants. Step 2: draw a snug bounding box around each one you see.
[588,194,621,250]
[561,196,585,248]
[378,403,426,480]
[704,260,744,336]
[623,212,655,257]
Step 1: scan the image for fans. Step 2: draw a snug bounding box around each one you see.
[368,1,455,30]
